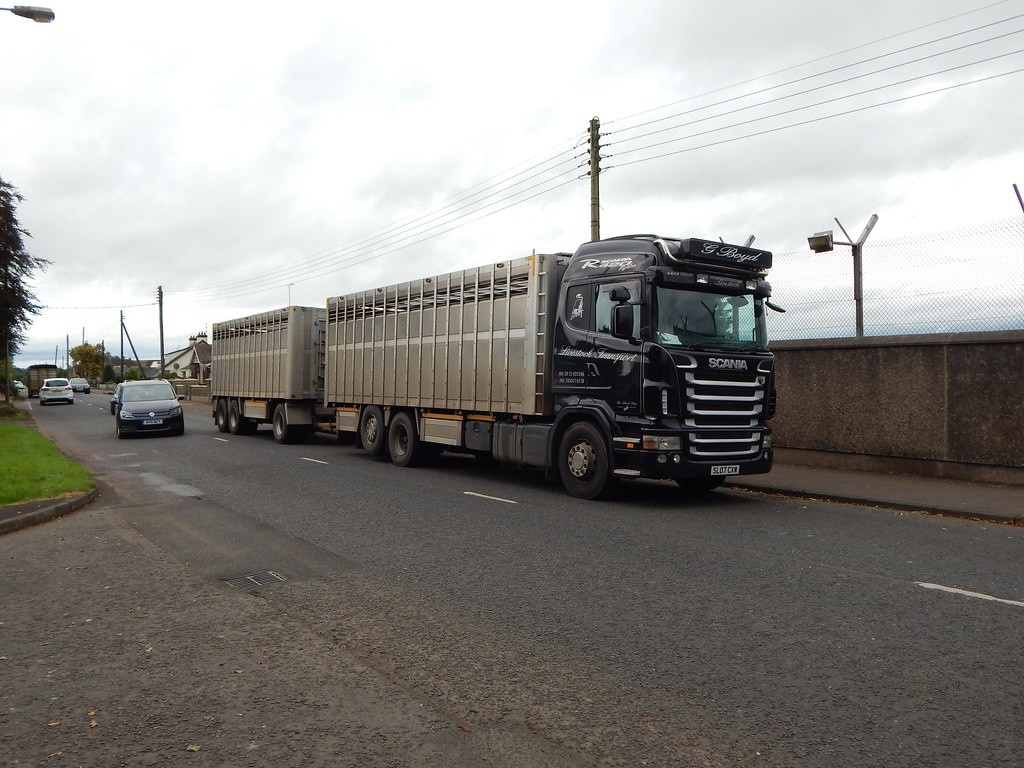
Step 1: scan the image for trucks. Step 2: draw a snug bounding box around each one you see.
[26,364,57,398]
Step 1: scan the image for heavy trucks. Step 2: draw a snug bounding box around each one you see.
[209,235,786,500]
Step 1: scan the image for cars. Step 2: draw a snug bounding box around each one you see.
[39,378,74,405]
[69,378,91,394]
[108,379,184,438]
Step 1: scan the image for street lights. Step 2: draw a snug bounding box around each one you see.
[806,213,879,336]
